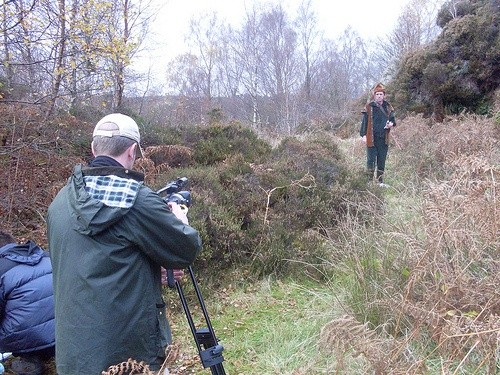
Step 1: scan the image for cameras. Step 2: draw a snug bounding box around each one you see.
[156,177,191,209]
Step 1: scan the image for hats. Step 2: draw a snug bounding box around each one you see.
[93,113,144,158]
[374,83,386,92]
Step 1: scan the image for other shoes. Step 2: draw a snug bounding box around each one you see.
[377,182,390,188]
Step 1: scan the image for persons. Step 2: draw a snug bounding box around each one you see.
[45,113,202,375]
[360,84,396,188]
[0,230,55,375]
